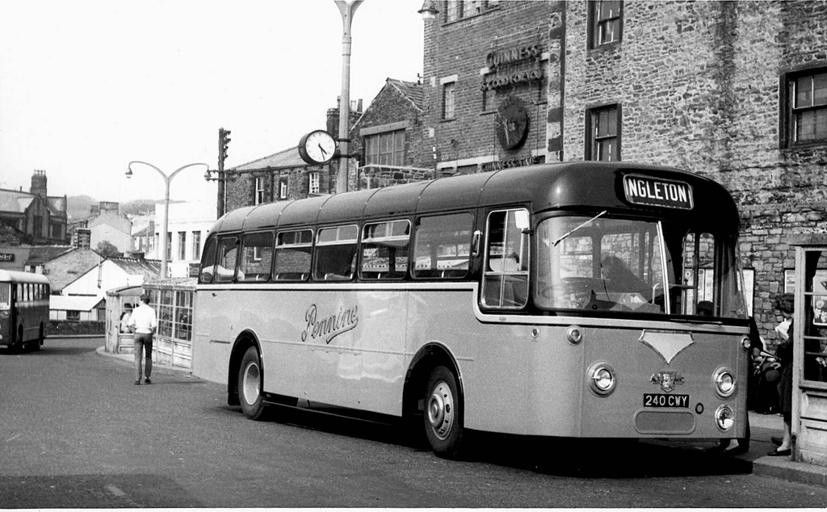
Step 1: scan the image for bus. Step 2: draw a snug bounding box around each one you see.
[0,269,50,354]
[190,162,754,455]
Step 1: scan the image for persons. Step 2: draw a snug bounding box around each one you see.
[120,303,139,333]
[128,294,157,384]
[766,293,821,456]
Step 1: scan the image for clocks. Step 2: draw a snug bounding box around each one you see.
[298,129,336,166]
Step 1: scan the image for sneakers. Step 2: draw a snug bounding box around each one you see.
[135,378,151,385]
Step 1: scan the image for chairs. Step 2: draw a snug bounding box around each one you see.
[601,255,654,302]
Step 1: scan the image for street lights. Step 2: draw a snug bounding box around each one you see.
[124,162,211,278]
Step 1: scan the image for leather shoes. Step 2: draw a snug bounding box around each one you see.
[767,437,790,456]
